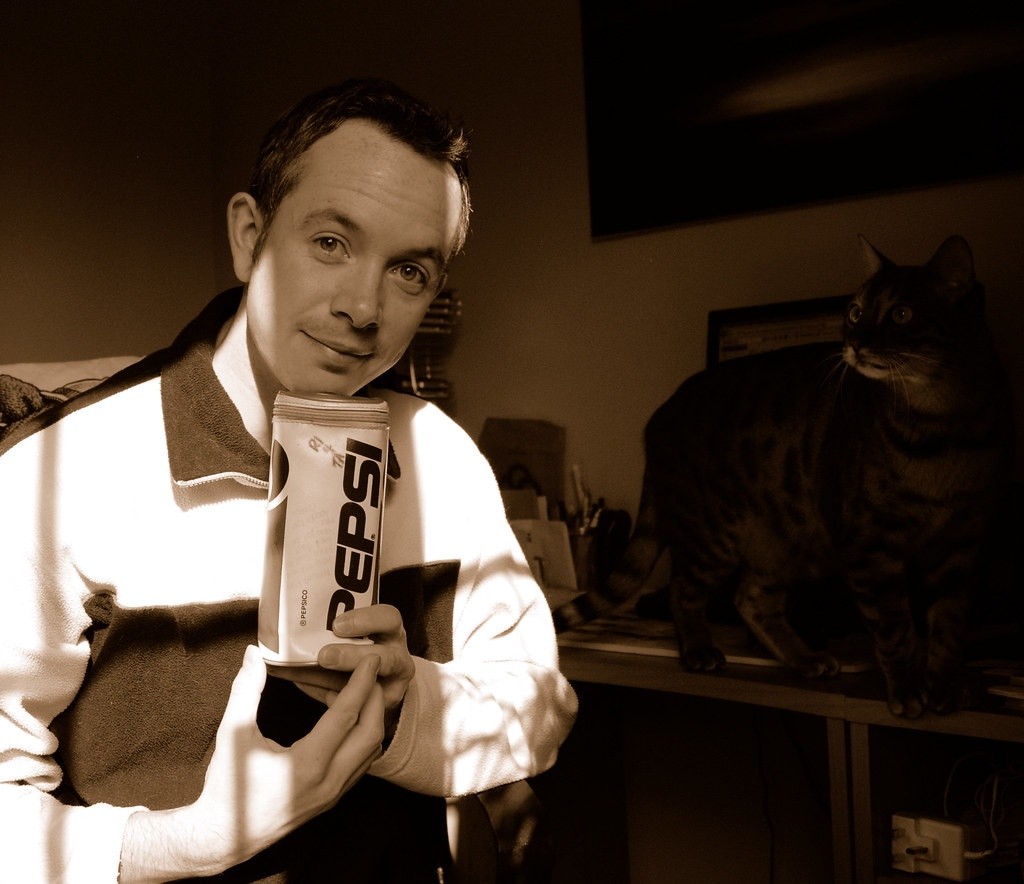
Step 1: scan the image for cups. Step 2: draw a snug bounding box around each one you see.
[257,388,391,666]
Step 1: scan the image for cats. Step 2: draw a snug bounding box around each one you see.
[580,233,1019,719]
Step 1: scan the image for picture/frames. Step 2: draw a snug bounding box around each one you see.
[579,0,1024,245]
[706,293,853,367]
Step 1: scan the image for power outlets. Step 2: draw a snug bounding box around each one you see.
[891,811,939,875]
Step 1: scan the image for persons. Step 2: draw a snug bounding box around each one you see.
[0,76,579,884]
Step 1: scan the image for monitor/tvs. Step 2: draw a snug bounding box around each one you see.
[578,0,1024,244]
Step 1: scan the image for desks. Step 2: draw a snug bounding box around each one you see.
[446,619,1023,884]
